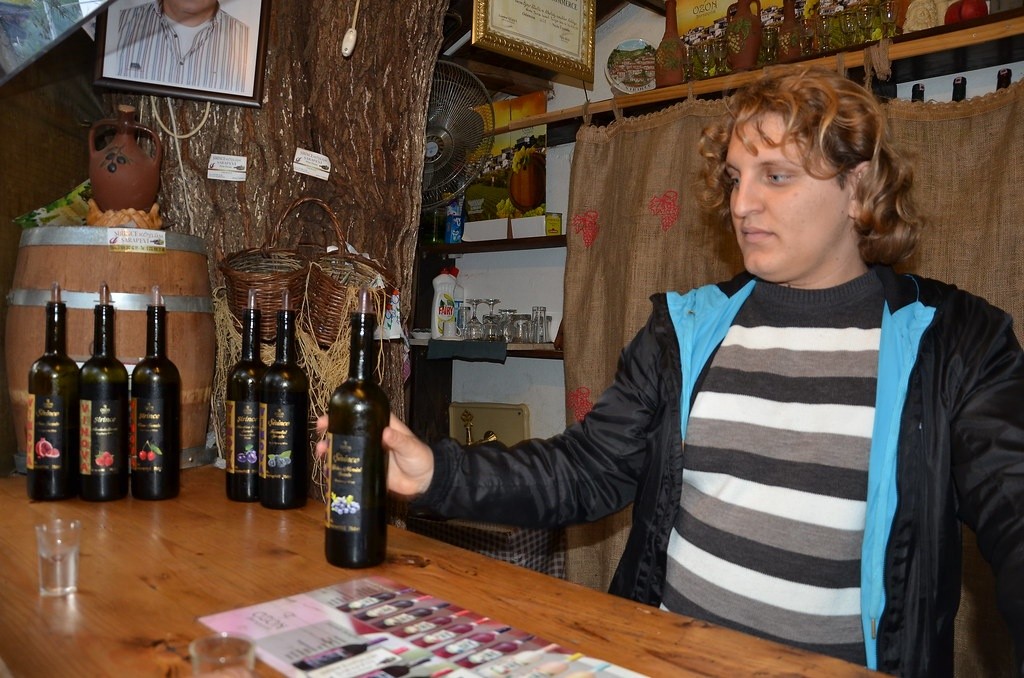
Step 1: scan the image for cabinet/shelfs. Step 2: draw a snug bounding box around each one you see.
[409,16,1024,351]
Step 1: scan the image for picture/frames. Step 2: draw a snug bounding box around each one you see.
[92,0,272,108]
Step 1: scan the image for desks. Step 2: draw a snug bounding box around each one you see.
[0,463,890,677]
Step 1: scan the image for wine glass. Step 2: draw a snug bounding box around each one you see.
[463,298,501,341]
[681,0,899,85]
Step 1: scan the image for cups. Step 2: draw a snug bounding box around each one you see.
[187,633,255,678]
[457,307,550,343]
[443,322,461,337]
[34,519,79,596]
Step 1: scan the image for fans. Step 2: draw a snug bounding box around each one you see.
[421,59,497,210]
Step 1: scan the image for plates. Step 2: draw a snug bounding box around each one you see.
[436,338,465,341]
[603,38,657,95]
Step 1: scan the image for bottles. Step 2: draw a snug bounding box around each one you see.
[225,287,269,503]
[912,83,925,103]
[129,284,182,502]
[952,77,967,103]
[654,0,687,88]
[258,290,309,511]
[25,281,79,501]
[778,0,801,61]
[75,281,130,503]
[996,68,1012,92]
[324,289,391,571]
[431,178,465,243]
[430,263,464,339]
[87,104,163,214]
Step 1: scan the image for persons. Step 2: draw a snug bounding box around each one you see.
[316,69,1024,678]
[114,0,248,93]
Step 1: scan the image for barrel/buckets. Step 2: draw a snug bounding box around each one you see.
[4,227,217,475]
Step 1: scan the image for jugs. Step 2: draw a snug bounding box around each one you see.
[725,0,762,72]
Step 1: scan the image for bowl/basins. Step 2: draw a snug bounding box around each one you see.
[410,331,431,340]
